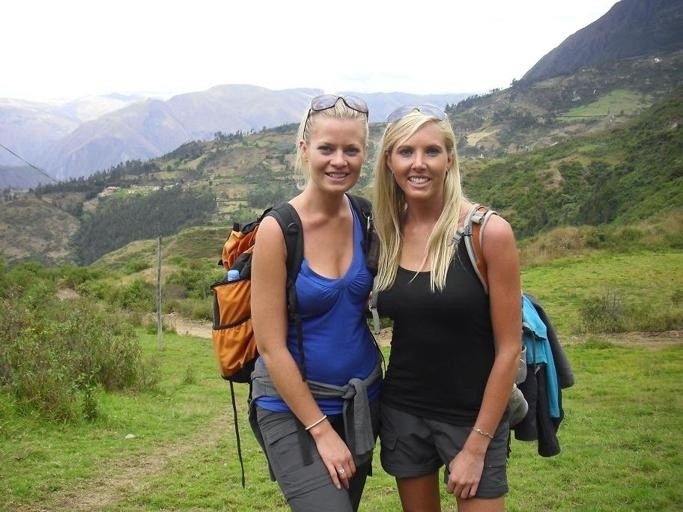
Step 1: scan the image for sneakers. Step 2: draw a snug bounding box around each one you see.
[518,345,530,388]
[509,383,528,429]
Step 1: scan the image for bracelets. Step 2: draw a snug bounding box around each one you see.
[473,426,492,440]
[304,415,329,430]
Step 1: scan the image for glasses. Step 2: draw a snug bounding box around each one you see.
[384,104,445,122]
[311,94,369,113]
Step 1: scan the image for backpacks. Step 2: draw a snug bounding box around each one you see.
[209,193,381,383]
[458,207,528,428]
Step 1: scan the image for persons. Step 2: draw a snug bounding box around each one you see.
[374,111,523,512]
[251,93,379,512]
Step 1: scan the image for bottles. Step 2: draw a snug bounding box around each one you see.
[227,270,239,282]
